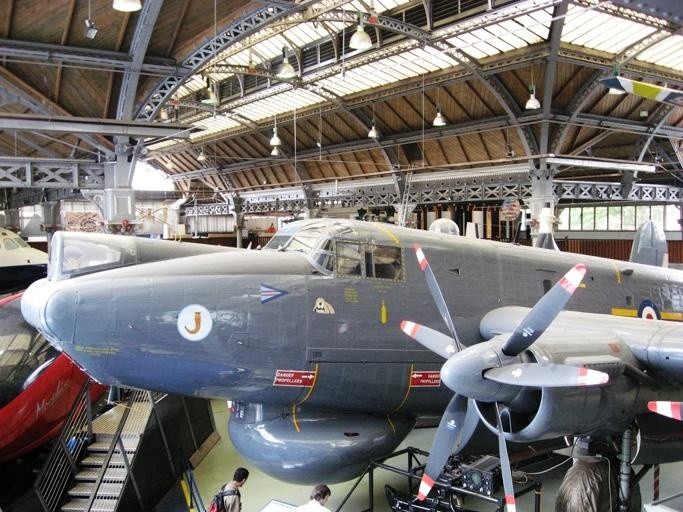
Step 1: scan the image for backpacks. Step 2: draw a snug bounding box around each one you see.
[208,483,241,512]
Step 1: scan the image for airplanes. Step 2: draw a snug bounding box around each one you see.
[1,227,110,464]
[18,217,681,508]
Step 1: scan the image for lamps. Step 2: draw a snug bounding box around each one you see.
[197,147,206,160]
[368,102,380,137]
[639,98,649,117]
[525,64,541,109]
[433,86,447,126]
[608,66,626,95]
[271,146,280,157]
[270,116,283,146]
[349,12,374,49]
[85,0,101,40]
[112,0,142,12]
[276,46,298,79]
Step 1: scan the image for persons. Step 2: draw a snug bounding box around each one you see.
[295,483,331,512]
[223,467,250,512]
